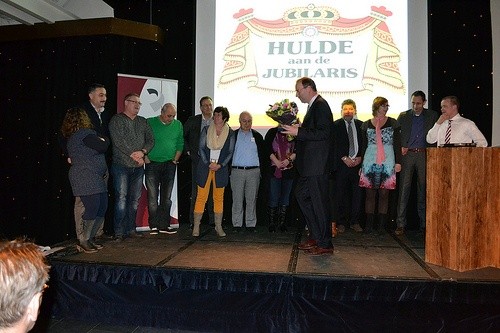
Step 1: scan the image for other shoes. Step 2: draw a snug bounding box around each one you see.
[77,242,98,254]
[115,235,124,241]
[245,227,256,232]
[159,228,178,234]
[305,230,312,237]
[350,224,362,232]
[394,226,403,236]
[297,239,320,249]
[235,227,242,232]
[128,232,136,238]
[335,225,346,233]
[150,227,159,235]
[303,245,333,256]
[89,237,104,250]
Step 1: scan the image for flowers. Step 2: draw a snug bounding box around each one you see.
[265,99,299,142]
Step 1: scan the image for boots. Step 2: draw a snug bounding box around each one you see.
[377,212,387,235]
[363,214,375,237]
[192,212,203,236]
[276,205,289,233]
[268,207,276,233]
[214,213,226,236]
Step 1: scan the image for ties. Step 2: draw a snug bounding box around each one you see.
[346,120,355,158]
[445,119,453,144]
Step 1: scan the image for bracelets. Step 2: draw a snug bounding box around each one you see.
[287,157,292,164]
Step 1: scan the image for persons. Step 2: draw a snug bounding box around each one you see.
[394,91,438,236]
[426,96,488,147]
[59,84,183,253]
[184,96,264,236]
[0,240,50,333]
[358,97,401,235]
[332,100,368,237]
[264,124,296,233]
[281,78,334,255]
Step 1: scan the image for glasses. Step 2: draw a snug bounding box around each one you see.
[202,104,214,107]
[297,84,309,94]
[125,99,142,107]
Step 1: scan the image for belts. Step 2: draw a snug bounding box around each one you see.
[408,148,425,154]
[232,166,259,170]
